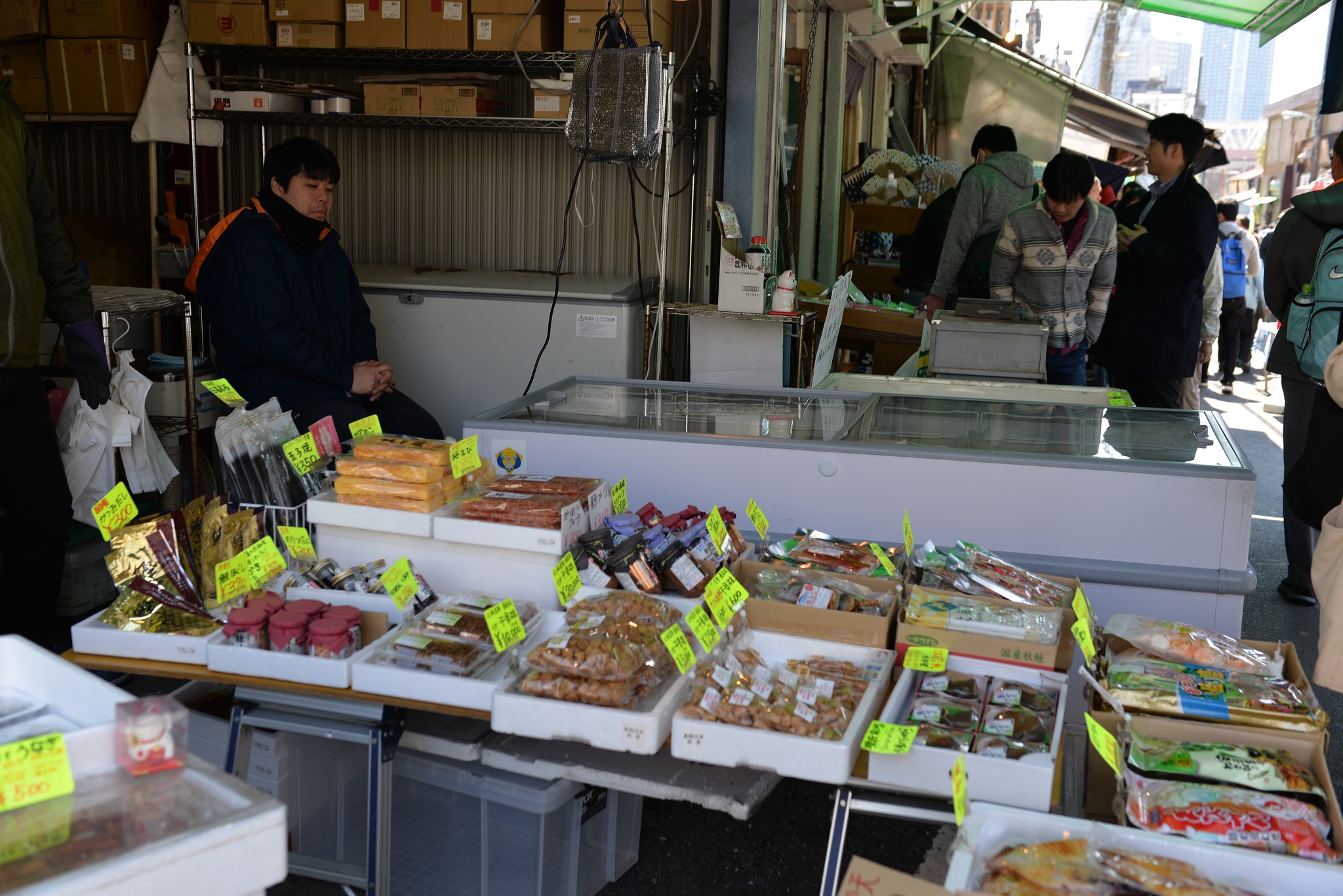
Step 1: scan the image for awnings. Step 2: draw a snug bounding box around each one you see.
[921,7,1222,180]
[1243,196,1278,206]
[1108,0,1330,48]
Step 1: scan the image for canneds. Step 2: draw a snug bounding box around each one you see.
[561,502,746,598]
[222,590,364,659]
[283,558,439,615]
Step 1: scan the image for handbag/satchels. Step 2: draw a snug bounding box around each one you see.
[957,229,1000,299]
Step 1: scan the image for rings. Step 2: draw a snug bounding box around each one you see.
[1126,232,1130,236]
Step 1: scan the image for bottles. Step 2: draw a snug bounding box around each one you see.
[745,236,772,288]
[224,597,364,660]
[561,513,747,598]
[1288,283,1315,345]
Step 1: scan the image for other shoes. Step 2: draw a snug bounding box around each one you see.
[1237,359,1250,371]
[1219,360,1224,369]
[1278,574,1317,605]
[1221,380,1233,394]
[1199,373,1208,387]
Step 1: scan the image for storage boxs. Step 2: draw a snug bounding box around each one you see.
[345,0,406,49]
[714,211,779,314]
[276,23,345,49]
[563,11,672,55]
[269,0,346,25]
[0,492,1343,895]
[534,88,570,119]
[564,0,672,25]
[46,0,161,40]
[46,39,162,116]
[210,89,305,113]
[406,0,471,51]
[471,15,561,52]
[61,212,152,349]
[363,83,502,117]
[0,0,50,114]
[470,0,560,22]
[187,1,276,47]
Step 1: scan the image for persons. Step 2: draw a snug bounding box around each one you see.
[916,124,1039,324]
[989,150,1118,387]
[1180,197,1292,413]
[1261,135,1343,604]
[1084,175,1150,229]
[177,137,444,503]
[1106,113,1220,407]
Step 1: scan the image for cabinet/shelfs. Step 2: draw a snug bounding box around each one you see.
[98,300,200,515]
[183,39,674,135]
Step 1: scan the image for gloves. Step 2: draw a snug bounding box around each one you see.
[62,319,112,410]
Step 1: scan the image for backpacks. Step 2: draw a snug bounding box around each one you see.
[1286,228,1343,380]
[1218,228,1246,299]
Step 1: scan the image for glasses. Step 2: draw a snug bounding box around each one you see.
[1329,151,1339,160]
